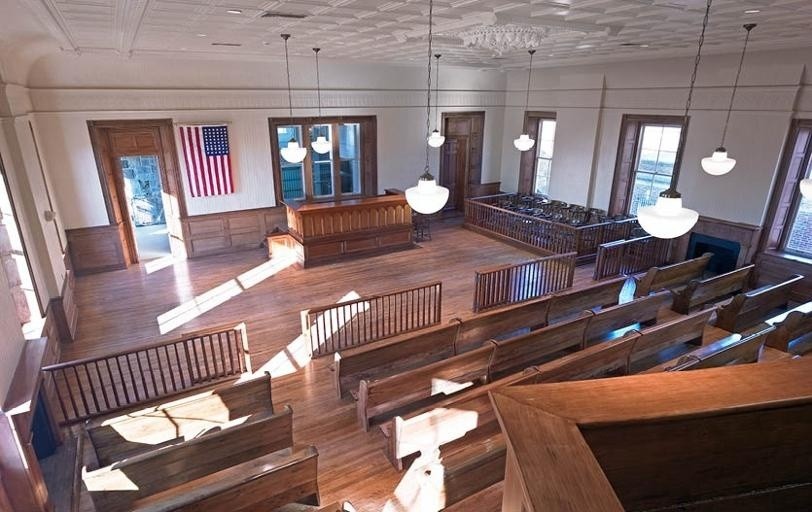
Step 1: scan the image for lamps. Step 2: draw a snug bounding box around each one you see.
[512,50,536,152]
[310,47,332,155]
[279,33,308,165]
[798,178,811,203]
[427,54,445,148]
[405,1,450,216]
[700,22,757,177]
[462,28,545,54]
[637,0,714,238]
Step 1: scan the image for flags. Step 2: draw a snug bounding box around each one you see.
[180,123,235,199]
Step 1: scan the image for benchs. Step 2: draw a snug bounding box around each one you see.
[84,405,293,511]
[351,290,672,431]
[414,320,777,512]
[711,274,804,323]
[327,276,627,400]
[763,301,810,351]
[380,304,720,473]
[668,262,754,313]
[84,370,274,467]
[132,442,319,511]
[632,253,713,297]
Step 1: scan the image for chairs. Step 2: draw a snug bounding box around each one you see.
[412,212,433,243]
[489,194,631,259]
